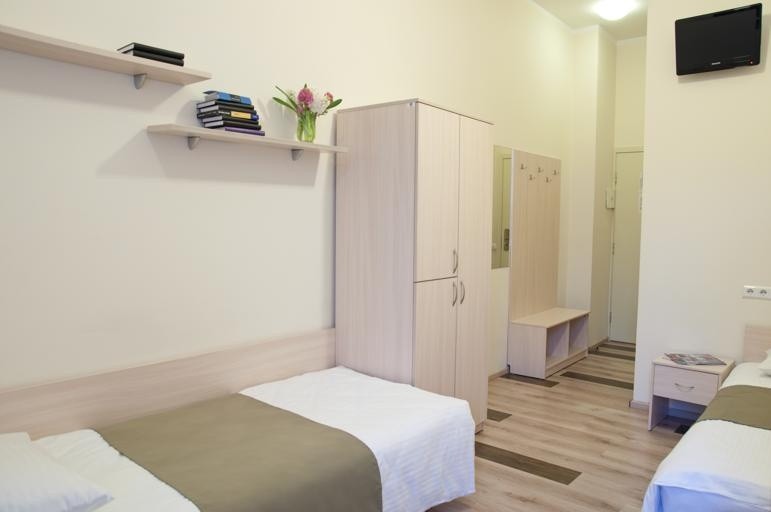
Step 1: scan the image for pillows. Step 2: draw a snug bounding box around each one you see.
[1,429,90,512]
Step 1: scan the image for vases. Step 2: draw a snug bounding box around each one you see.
[296,112,316,142]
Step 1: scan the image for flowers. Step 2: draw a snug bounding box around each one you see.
[272,83,343,141]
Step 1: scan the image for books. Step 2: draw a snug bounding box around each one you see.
[124,50,185,67]
[117,42,185,60]
[195,90,265,136]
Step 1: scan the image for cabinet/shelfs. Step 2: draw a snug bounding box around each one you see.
[336,99,493,434]
[508,306,590,380]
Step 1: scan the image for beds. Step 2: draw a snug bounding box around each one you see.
[658,320,771,512]
[32,366,476,512]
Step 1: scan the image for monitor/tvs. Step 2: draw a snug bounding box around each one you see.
[674,2,764,76]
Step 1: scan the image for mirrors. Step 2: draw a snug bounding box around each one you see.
[493,145,512,270]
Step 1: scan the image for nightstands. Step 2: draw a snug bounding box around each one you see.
[649,352,735,431]
